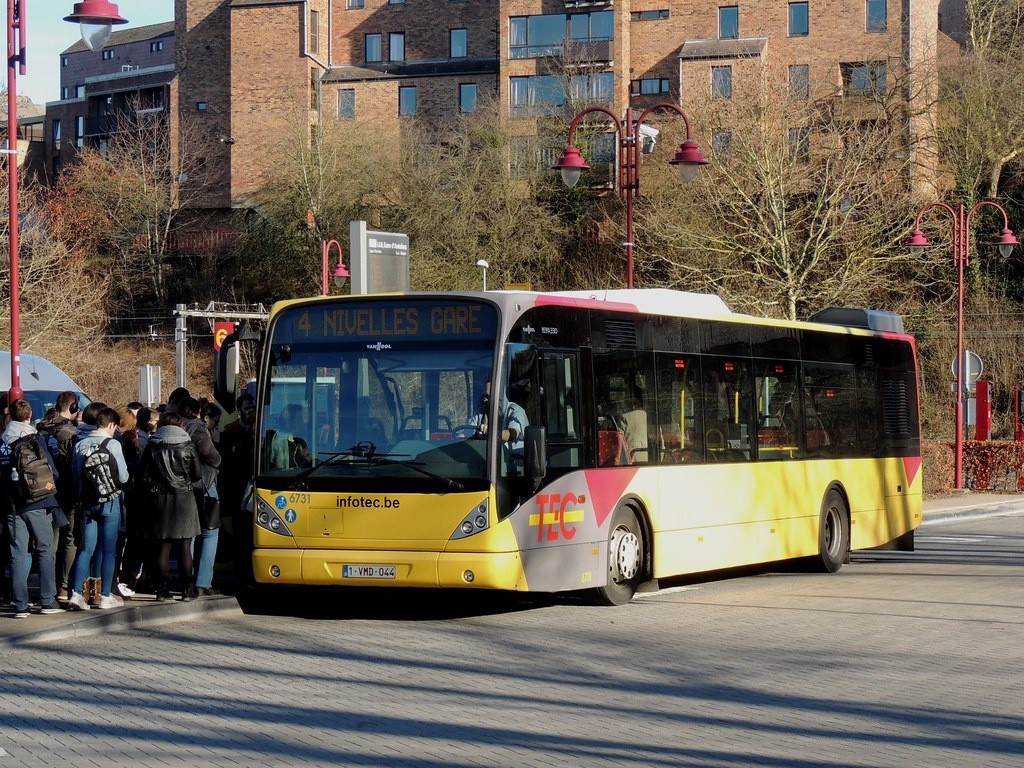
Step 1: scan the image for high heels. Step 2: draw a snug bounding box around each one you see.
[196,585,221,596]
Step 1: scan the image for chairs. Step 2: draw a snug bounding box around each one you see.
[401,413,845,470]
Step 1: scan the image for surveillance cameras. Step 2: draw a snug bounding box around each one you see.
[635,122,659,138]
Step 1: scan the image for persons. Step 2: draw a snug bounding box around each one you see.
[456,376,530,459]
[1,386,391,619]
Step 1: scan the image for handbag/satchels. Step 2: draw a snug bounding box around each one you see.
[194,478,222,530]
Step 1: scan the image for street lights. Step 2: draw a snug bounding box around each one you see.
[552,102,709,287]
[903,200,1020,488]
[5,0,129,405]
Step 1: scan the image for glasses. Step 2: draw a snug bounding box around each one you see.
[207,415,220,425]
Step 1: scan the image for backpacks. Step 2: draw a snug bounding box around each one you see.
[11,430,57,504]
[83,437,124,504]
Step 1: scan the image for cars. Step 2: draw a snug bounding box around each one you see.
[0,351,94,427]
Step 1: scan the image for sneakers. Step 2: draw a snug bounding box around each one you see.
[98,593,124,609]
[68,588,90,611]
[41,596,69,614]
[15,606,31,619]
[112,583,135,597]
[56,588,68,600]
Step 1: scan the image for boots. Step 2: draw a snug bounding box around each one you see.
[81,577,91,604]
[89,576,123,605]
[156,577,173,602]
[182,577,198,602]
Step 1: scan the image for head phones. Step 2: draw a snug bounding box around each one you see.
[69,391,78,414]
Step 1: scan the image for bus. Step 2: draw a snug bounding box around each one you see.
[208,283,922,606]
